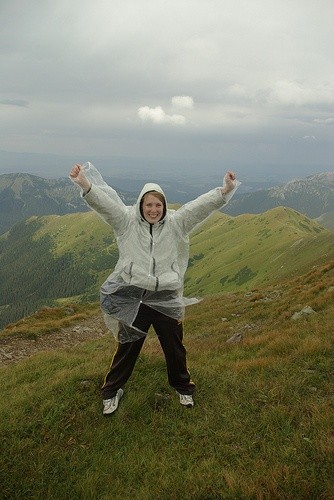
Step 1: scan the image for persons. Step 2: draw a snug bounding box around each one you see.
[68,161,241,418]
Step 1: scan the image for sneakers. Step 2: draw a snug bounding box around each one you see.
[102,388,124,415]
[176,390,194,407]
